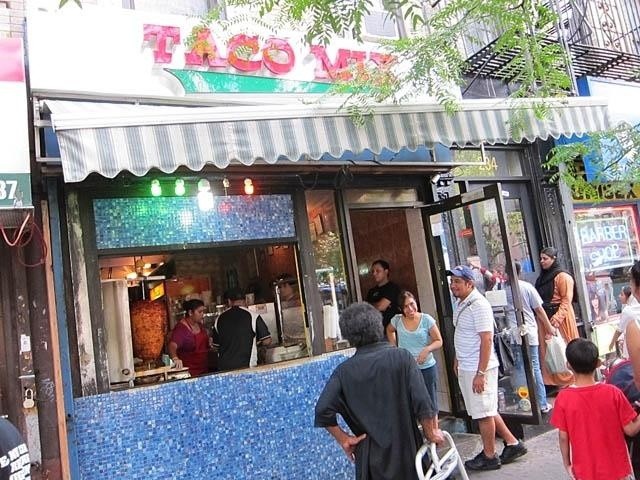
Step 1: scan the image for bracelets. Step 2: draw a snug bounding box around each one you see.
[173,357,178,362]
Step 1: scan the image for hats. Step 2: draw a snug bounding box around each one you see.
[446,265,476,281]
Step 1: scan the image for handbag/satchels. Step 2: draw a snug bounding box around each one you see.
[492,333,517,375]
[545,328,567,374]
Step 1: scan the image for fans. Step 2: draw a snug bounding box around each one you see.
[101,256,166,281]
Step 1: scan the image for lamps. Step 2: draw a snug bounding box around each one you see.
[150,176,253,212]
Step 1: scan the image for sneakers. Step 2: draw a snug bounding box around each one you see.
[542,403,552,413]
[465,451,501,469]
[499,438,528,464]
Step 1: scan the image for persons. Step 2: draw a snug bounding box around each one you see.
[386,290,443,443]
[313,302,445,480]
[167,299,214,376]
[212,288,271,372]
[271,274,300,311]
[0,409,31,480]
[445,247,640,479]
[365,261,406,340]
